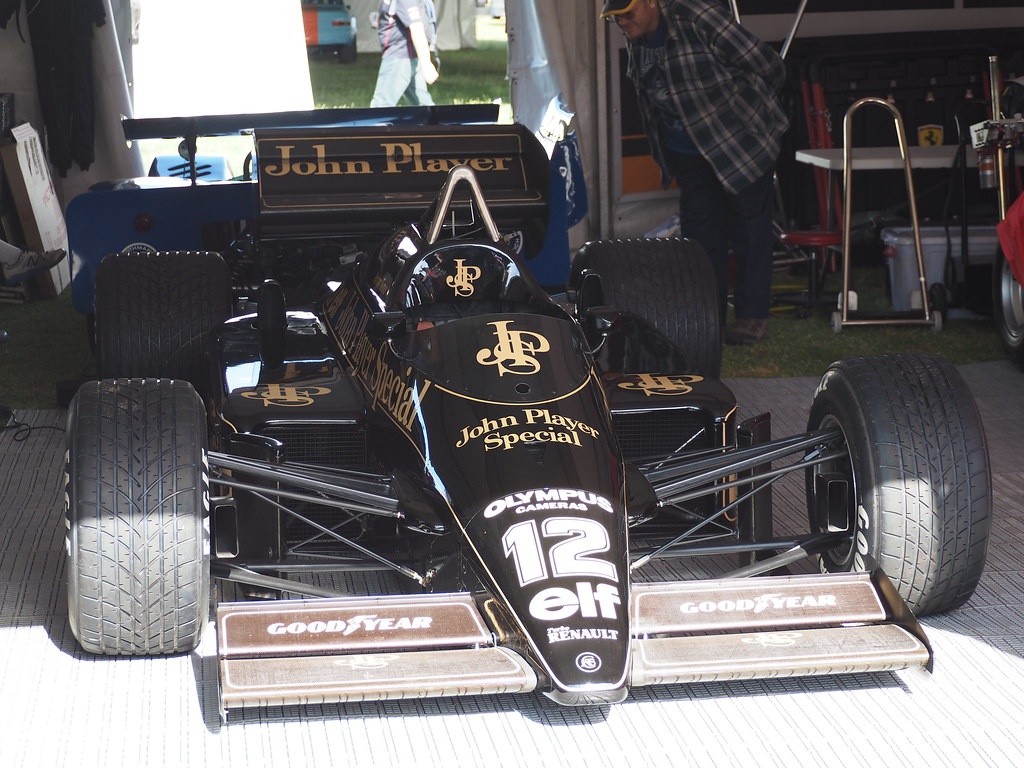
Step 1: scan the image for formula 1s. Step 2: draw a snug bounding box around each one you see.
[62,104,992,722]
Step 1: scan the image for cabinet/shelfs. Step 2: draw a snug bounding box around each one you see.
[597,0,1024,240]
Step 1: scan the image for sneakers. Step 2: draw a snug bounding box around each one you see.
[724,316,767,346]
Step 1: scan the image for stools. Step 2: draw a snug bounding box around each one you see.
[780,231,842,318]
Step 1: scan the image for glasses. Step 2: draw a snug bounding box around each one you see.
[605,7,636,21]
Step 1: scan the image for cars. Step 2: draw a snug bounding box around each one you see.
[301,0,357,61]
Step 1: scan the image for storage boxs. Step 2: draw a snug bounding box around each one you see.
[880,227,999,312]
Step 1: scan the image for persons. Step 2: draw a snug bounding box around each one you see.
[599,0,795,344]
[365,0,440,113]
[0,238,68,344]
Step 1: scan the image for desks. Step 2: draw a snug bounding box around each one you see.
[796,145,1024,311]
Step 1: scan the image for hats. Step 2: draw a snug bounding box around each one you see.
[600,0,636,16]
